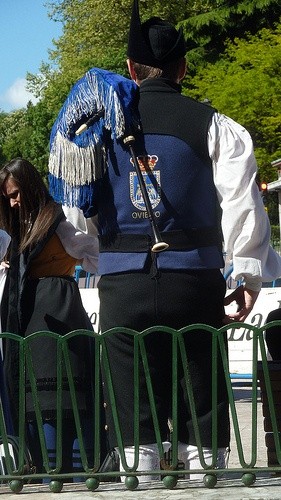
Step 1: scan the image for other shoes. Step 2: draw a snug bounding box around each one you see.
[115,442,172,483]
[178,441,230,480]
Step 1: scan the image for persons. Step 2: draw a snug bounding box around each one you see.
[48,16,281,482]
[0,158,100,484]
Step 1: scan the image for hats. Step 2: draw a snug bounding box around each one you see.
[127,0,184,64]
